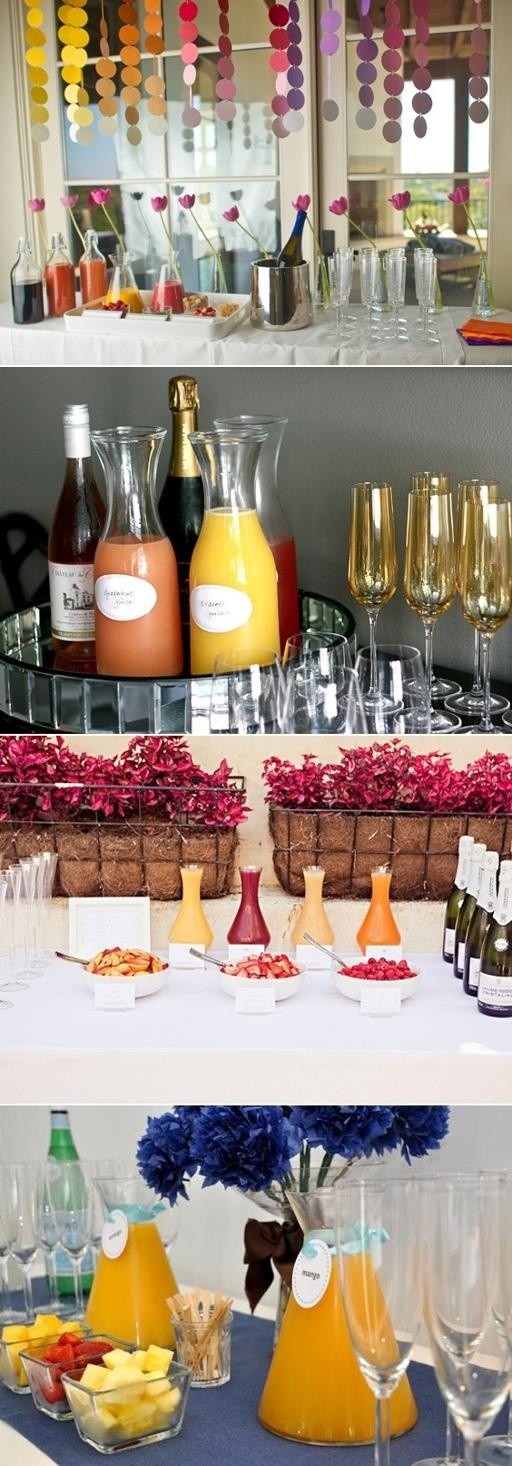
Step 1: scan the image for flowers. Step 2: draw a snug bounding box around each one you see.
[135,1106,452,1218]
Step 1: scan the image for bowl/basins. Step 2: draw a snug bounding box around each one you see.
[219,963,309,1004]
[78,953,171,996]
[335,966,424,1004]
[1,1322,198,1457]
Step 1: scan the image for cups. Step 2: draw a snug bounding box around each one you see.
[249,256,315,333]
[168,1308,233,1389]
[204,625,434,733]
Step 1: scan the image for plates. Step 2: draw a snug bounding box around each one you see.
[61,285,252,342]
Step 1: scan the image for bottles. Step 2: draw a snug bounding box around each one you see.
[256,1185,422,1448]
[273,209,309,267]
[442,834,512,1023]
[79,1174,189,1350]
[8,234,44,325]
[44,232,76,319]
[37,1111,99,1295]
[46,373,304,682]
[166,862,403,958]
[103,252,145,313]
[78,228,108,310]
[149,248,186,314]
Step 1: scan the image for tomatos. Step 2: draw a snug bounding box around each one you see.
[29,1331,113,1404]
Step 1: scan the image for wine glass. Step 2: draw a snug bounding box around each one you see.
[0,849,61,1011]
[331,1165,512,1466]
[342,466,512,736]
[325,246,442,347]
[0,1157,100,1325]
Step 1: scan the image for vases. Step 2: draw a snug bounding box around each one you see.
[470,253,497,318]
